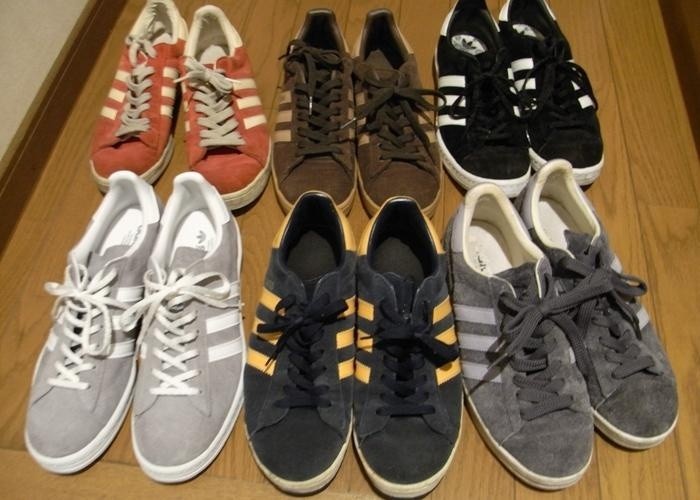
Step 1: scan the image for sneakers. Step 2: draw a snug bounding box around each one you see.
[352,8,444,219]
[90,1,189,195]
[24,169,166,475]
[499,0,604,187]
[513,158,681,451]
[240,190,356,495]
[130,172,242,484]
[435,0,531,199]
[353,195,465,499]
[445,182,594,491]
[269,9,358,217]
[172,5,270,211]
